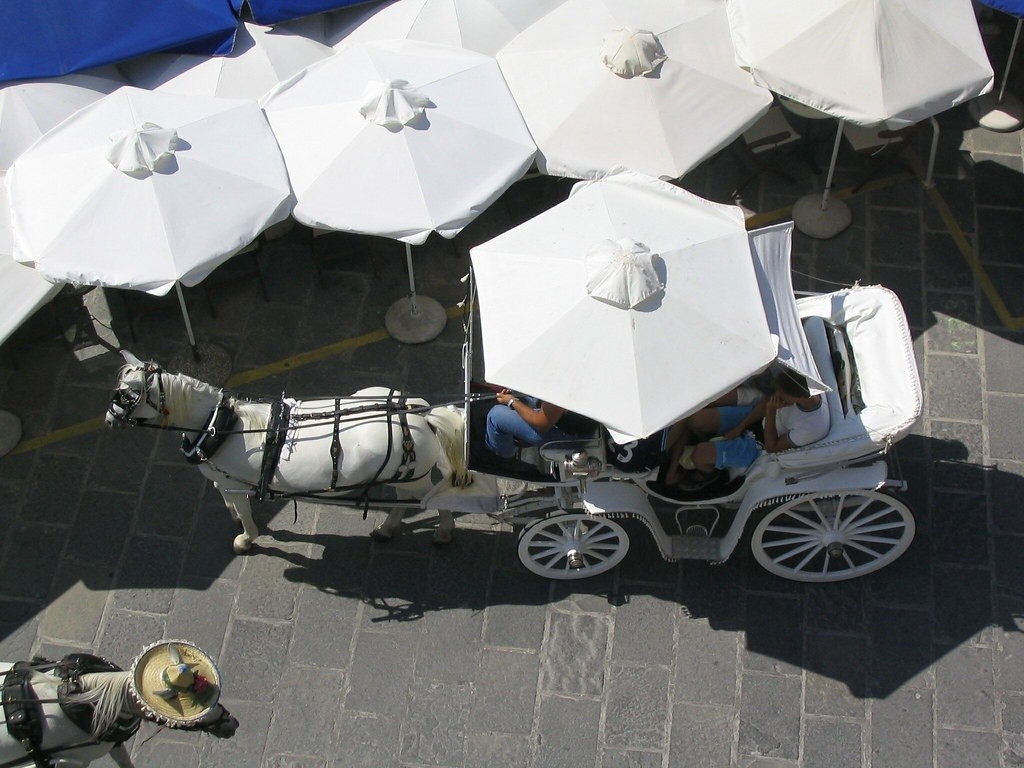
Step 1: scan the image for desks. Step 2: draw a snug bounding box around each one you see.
[776,92,837,177]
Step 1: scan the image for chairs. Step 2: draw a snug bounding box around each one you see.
[117,213,384,345]
[832,115,931,196]
[708,102,803,200]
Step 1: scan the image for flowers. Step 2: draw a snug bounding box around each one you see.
[195,674,209,694]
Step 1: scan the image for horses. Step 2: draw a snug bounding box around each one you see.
[104,350,482,554]
[0,662,239,768]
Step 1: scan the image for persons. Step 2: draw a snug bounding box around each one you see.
[665,368,830,491]
[485,389,569,463]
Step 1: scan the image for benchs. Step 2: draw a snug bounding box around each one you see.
[520,420,608,472]
[728,315,845,482]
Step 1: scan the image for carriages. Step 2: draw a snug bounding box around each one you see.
[102,169,924,586]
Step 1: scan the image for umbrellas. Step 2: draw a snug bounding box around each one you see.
[1,2,1023,445]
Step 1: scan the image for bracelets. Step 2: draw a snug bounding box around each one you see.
[508,398,520,410]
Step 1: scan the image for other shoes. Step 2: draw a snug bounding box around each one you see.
[646,480,679,496]
[691,470,715,483]
[484,449,517,465]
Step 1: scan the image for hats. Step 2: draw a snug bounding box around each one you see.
[128,639,223,728]
